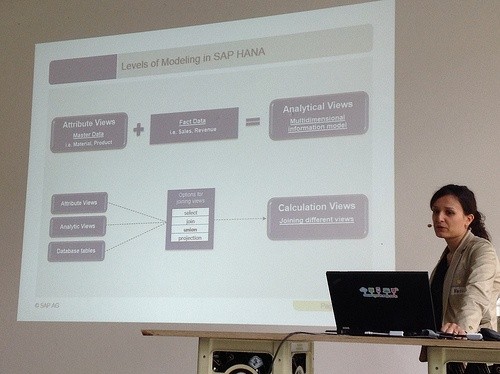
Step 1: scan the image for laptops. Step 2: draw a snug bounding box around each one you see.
[326,271,441,338]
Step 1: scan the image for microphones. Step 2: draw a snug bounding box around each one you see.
[428,224,432,227]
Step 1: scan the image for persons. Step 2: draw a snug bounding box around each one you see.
[418,184,500,374]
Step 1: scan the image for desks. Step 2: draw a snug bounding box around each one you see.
[141,329,500,374]
